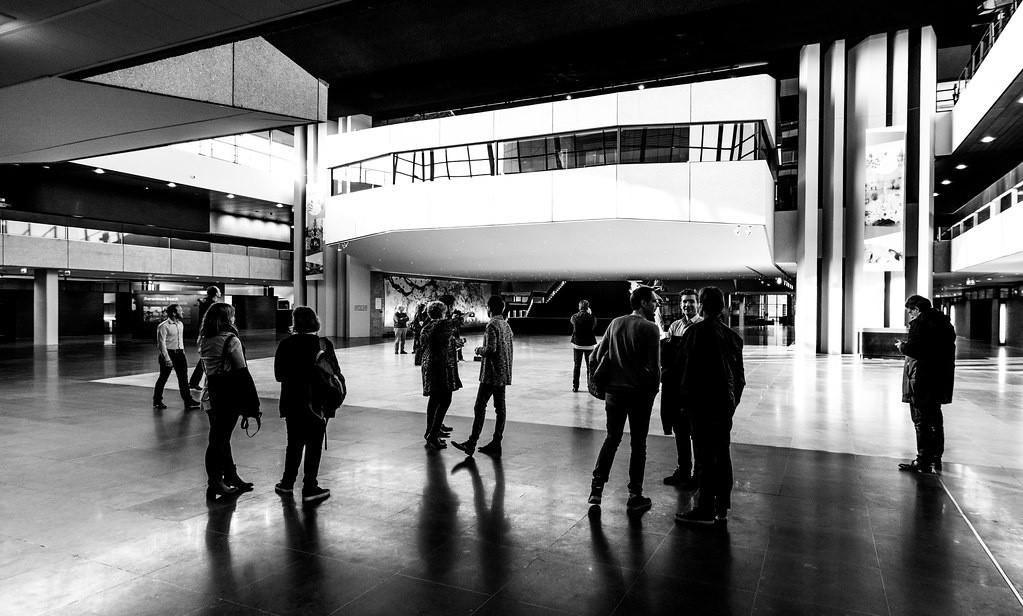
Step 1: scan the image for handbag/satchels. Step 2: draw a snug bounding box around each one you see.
[207,333,260,417]
[305,337,346,419]
[415,348,423,366]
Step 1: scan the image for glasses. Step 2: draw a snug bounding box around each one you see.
[908,309,913,315]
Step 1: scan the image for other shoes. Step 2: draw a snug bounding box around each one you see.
[275,481,293,492]
[715,504,728,520]
[437,429,450,436]
[184,399,200,407]
[589,488,602,505]
[627,495,651,510]
[303,487,330,501]
[899,455,942,474]
[663,474,691,486]
[440,424,453,431]
[450,440,477,455]
[153,401,167,409]
[573,387,579,392]
[674,507,715,524]
[677,477,698,491]
[188,383,202,391]
[478,438,502,453]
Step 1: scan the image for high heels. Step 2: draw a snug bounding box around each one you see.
[223,465,253,487]
[423,433,446,448]
[205,480,238,499]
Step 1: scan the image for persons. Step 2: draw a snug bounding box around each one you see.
[675,285,745,525]
[570,300,597,394]
[422,303,464,450]
[152,304,200,409]
[452,295,513,457]
[394,303,467,362]
[588,287,660,508]
[895,294,957,472]
[189,286,222,390]
[275,307,347,501]
[660,288,704,489]
[201,303,263,499]
